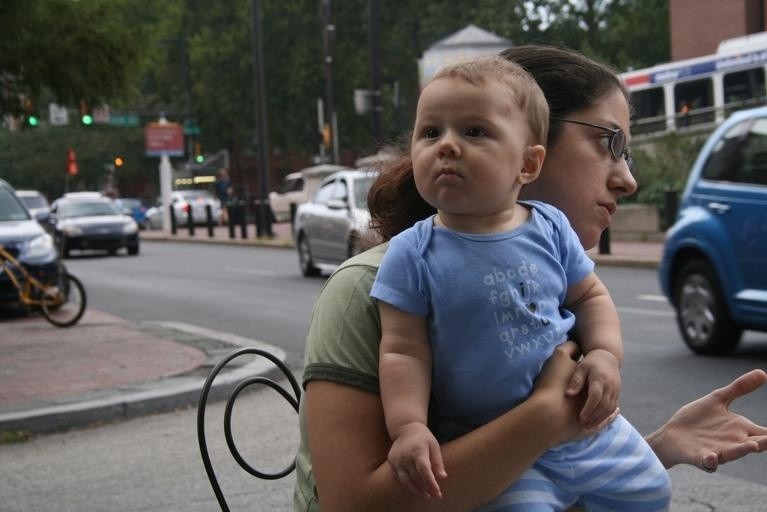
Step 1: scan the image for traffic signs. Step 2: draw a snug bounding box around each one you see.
[111,114,142,127]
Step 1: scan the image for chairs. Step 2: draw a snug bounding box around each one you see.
[196,346,302,512]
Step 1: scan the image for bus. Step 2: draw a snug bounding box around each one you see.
[617,30,767,141]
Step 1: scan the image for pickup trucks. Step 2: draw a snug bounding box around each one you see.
[266,164,349,225]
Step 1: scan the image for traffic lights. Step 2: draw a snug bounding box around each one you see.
[193,143,204,165]
[79,100,95,128]
[24,110,40,131]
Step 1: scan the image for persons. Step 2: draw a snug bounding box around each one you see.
[215,168,234,224]
[291,46,767,512]
[370,55,672,512]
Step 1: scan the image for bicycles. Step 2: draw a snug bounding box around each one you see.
[0,242,90,337]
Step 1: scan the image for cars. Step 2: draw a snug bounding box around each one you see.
[0,175,74,315]
[46,189,142,263]
[142,188,225,230]
[655,99,767,363]
[291,166,387,281]
[12,185,52,228]
[111,194,145,229]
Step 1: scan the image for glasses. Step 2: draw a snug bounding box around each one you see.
[550,108,632,162]
